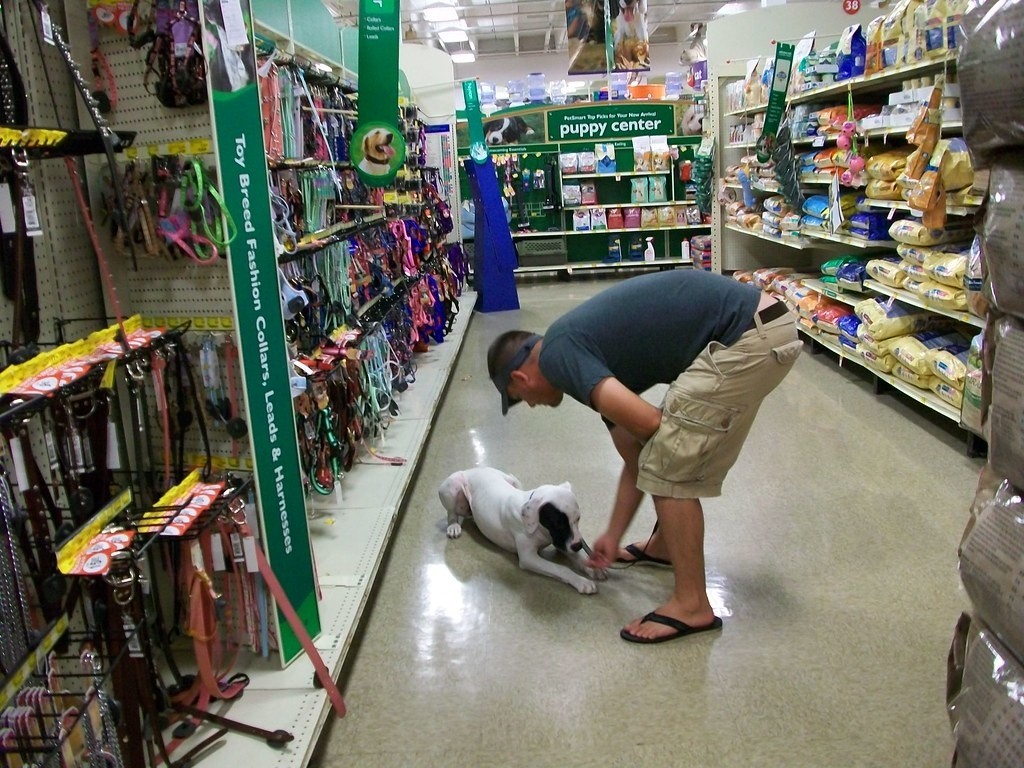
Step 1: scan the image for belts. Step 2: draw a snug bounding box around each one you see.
[1,2,346,768]
[746,302,789,332]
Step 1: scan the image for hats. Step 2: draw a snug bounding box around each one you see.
[491,334,543,416]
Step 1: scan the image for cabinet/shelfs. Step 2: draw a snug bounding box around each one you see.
[710,65,990,446]
[90,17,420,501]
[454,87,707,276]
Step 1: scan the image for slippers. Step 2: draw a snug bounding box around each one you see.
[620,604,723,645]
[615,545,674,567]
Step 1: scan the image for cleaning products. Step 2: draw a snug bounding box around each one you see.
[681,238,689,259]
[643,237,655,261]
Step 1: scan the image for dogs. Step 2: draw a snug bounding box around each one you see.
[609,0,651,70]
[483,115,536,146]
[680,104,705,135]
[438,467,610,595]
[357,128,395,176]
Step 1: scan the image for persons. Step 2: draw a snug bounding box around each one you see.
[487,268,805,645]
